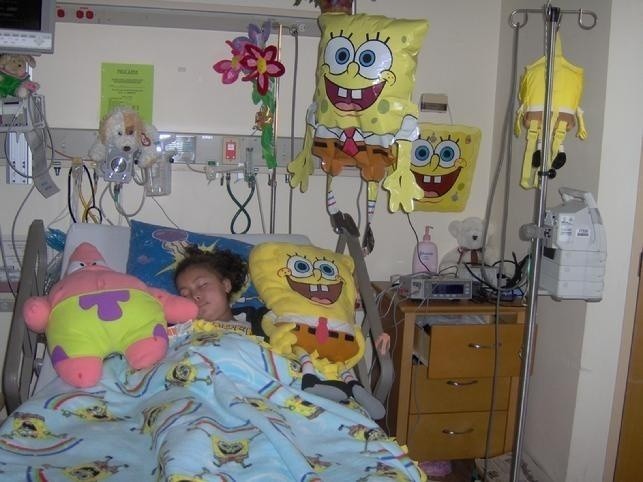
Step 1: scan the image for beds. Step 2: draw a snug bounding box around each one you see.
[0,219,427,480]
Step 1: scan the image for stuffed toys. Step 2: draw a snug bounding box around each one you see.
[1,53,40,100]
[23,243,199,390]
[88,107,160,168]
[441,218,502,279]
[248,241,386,419]
[513,31,585,190]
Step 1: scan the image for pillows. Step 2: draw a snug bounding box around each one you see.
[127,219,265,311]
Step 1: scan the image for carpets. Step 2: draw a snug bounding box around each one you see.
[417,449,536,482]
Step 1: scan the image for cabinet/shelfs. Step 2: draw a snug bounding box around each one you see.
[370,280,537,462]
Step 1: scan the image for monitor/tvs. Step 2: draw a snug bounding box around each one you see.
[0,0,55,55]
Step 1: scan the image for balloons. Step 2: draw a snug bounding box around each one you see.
[285,11,425,213]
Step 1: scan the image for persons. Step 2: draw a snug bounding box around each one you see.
[174,243,271,348]
